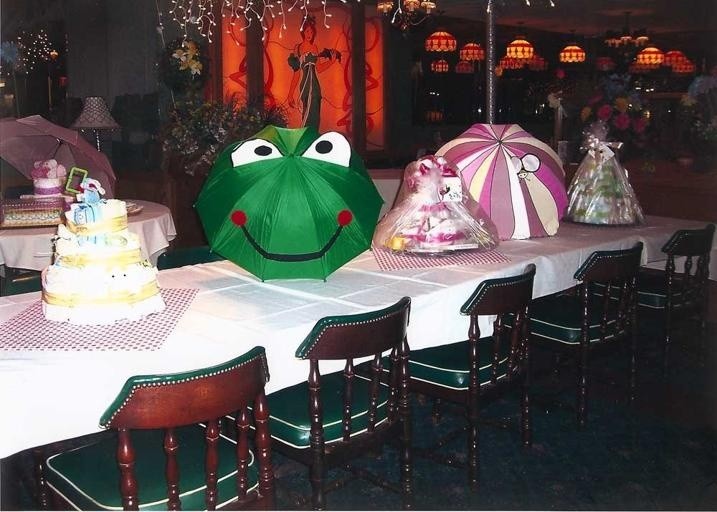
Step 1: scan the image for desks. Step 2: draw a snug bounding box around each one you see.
[0,214,715,463]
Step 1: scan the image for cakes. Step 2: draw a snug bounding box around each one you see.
[31,160,67,196]
[41,178,166,326]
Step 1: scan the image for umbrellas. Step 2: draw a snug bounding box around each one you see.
[193,124,386,285]
[433,122,570,242]
[0,113,117,199]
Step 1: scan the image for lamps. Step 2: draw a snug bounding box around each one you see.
[69,96,121,153]
[376,0,437,33]
[423,25,696,122]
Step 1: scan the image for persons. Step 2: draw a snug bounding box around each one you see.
[287,14,338,133]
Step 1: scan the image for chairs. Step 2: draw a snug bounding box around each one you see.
[22,346,277,511]
[354,263,530,495]
[592,224,715,384]
[226,295,415,511]
[504,241,644,429]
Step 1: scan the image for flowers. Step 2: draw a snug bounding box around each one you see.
[153,40,284,174]
